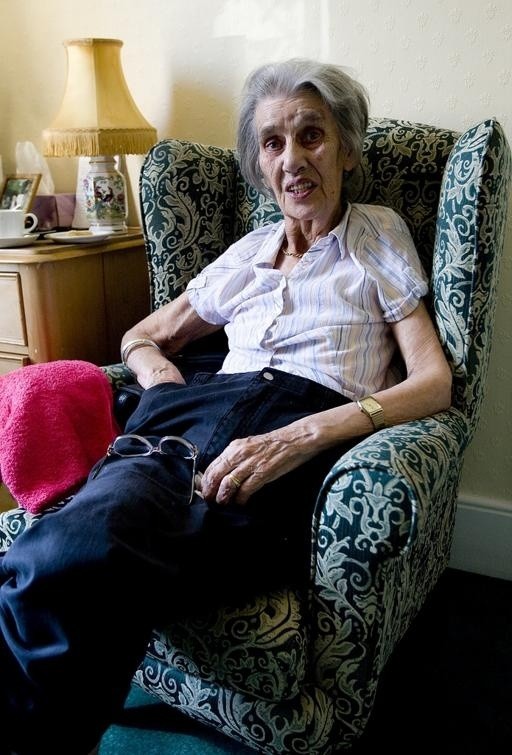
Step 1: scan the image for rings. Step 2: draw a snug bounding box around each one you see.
[228,475,241,487]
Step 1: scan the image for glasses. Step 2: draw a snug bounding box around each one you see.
[92,434,199,505]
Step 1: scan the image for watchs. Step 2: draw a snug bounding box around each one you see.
[356,395,384,431]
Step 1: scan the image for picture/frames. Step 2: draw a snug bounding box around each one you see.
[2,171,42,217]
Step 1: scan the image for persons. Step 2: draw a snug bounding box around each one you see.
[8,192,21,210]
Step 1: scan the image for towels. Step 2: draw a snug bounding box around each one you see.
[0,358,120,516]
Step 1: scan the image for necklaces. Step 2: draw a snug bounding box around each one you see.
[280,245,304,258]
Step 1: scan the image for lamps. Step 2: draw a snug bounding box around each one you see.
[41,32,158,237]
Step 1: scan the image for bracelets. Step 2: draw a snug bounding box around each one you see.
[122,339,160,365]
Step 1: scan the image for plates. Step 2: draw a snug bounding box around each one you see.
[46,230,114,243]
[0,235,41,247]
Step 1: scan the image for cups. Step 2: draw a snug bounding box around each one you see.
[0,209,37,238]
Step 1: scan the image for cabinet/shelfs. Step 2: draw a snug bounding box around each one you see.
[1,223,153,383]
